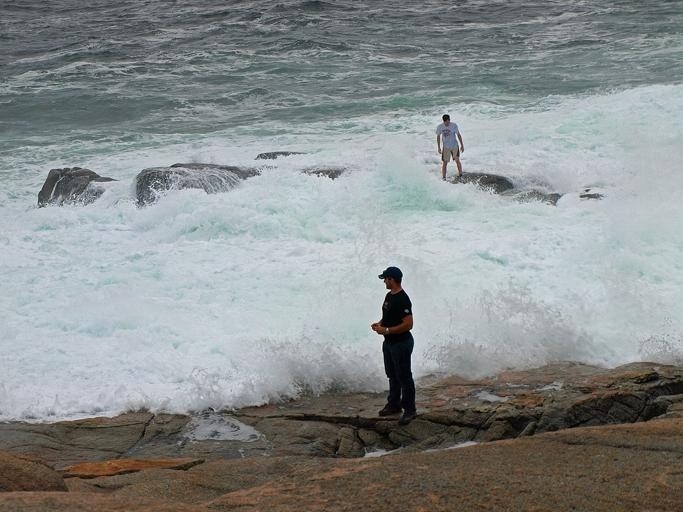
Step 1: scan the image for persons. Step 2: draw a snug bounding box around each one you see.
[371,267,417,426]
[435,114,464,181]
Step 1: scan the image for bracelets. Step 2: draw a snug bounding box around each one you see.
[385,328,389,335]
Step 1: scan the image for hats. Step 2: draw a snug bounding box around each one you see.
[377,267,402,279]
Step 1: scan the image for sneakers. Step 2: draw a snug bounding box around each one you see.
[398,410,417,425]
[378,403,402,416]
